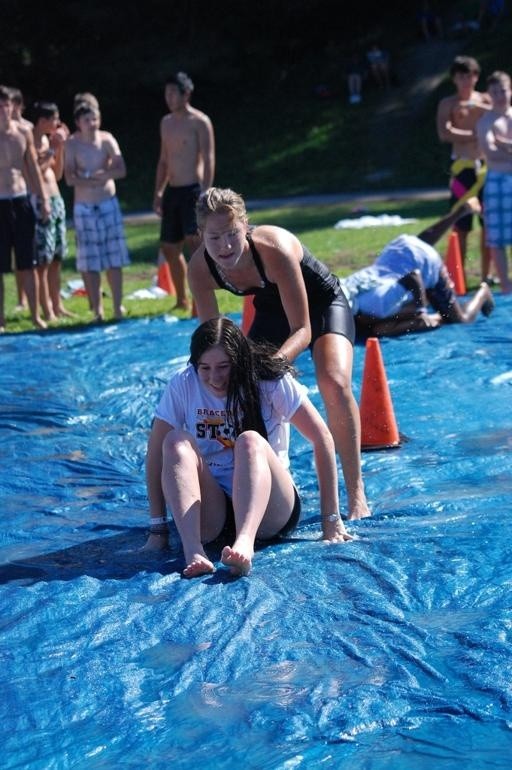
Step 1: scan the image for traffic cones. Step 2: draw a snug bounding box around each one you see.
[242,293,256,337]
[358,338,407,451]
[157,264,175,294]
[445,232,469,297]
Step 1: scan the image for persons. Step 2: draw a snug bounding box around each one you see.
[0,85,50,334]
[63,101,130,322]
[185,188,371,521]
[475,71,512,292]
[354,304,444,339]
[436,55,500,286]
[151,71,216,315]
[340,45,392,105]
[23,100,79,323]
[140,315,355,578]
[9,87,34,311]
[338,195,495,329]
[73,92,100,310]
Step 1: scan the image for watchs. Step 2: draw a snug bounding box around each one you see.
[321,513,342,523]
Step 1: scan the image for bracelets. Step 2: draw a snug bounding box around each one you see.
[85,169,90,179]
[148,527,170,535]
[415,307,427,314]
[148,516,169,526]
